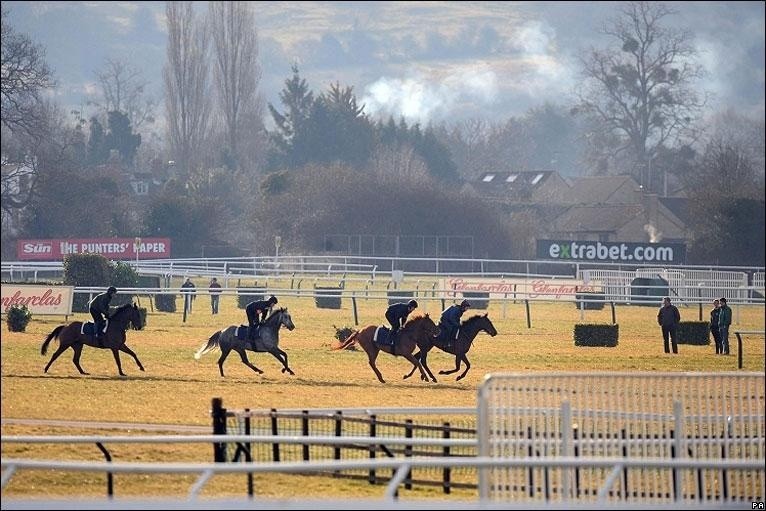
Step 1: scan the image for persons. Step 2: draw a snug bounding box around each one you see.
[246,296,278,343]
[656,297,680,353]
[709,299,723,354]
[718,298,731,354]
[384,300,418,344]
[208,277,222,314]
[180,278,196,313]
[439,299,471,348]
[89,286,117,338]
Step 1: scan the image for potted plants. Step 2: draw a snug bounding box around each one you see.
[4,304,32,333]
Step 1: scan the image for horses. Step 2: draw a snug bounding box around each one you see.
[194,306,295,377]
[403,312,497,381]
[330,313,441,384]
[41,302,144,376]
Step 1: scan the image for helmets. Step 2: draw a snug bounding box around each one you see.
[409,300,417,308]
[270,297,278,304]
[108,287,117,295]
[461,300,471,307]
